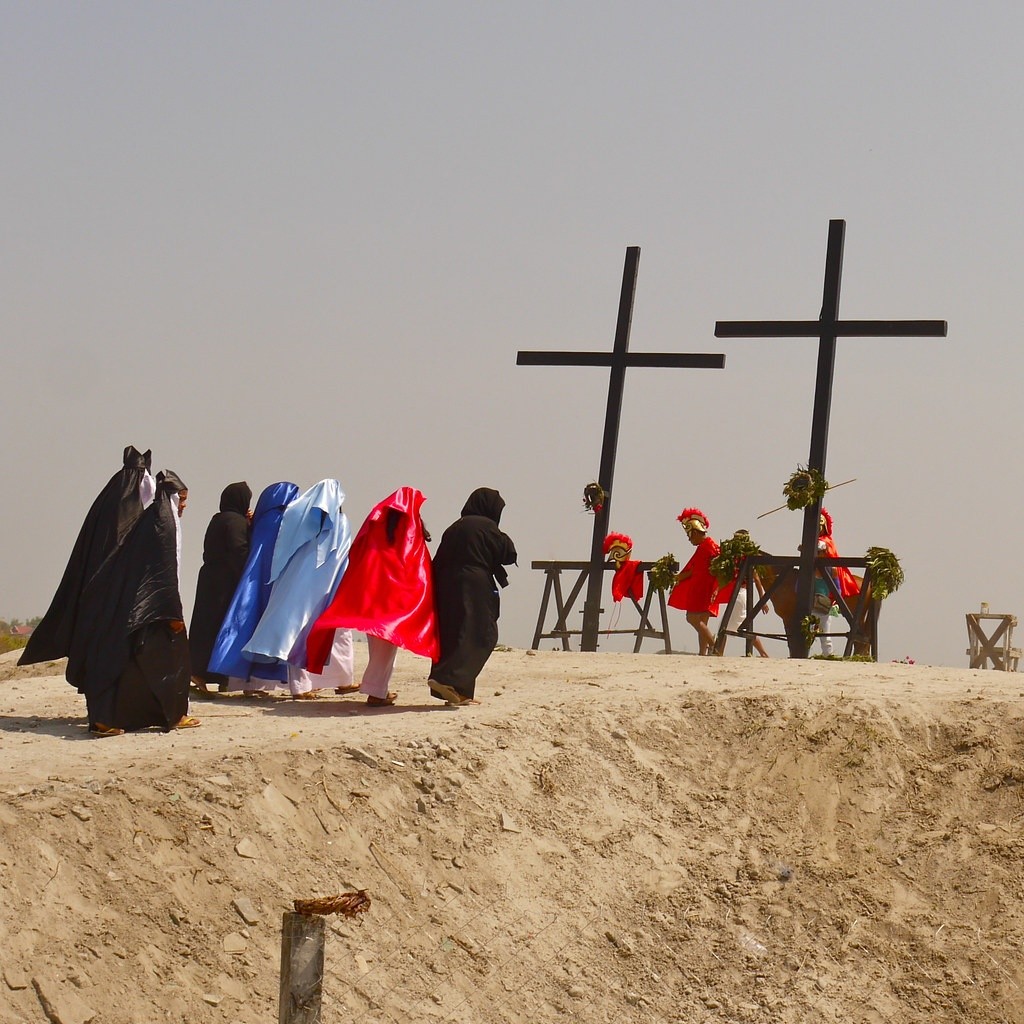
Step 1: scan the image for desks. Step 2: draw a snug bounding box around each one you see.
[965,611,1023,673]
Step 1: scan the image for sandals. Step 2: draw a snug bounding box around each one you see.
[303,692,320,698]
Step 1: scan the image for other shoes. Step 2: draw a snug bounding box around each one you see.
[387,692,397,699]
[293,695,316,700]
[367,698,397,707]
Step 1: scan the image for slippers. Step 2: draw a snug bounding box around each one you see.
[335,683,360,694]
[427,679,460,703]
[171,717,201,728]
[245,691,268,698]
[189,683,216,701]
[444,698,480,706]
[90,726,124,736]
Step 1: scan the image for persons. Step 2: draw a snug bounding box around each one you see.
[718,529,770,657]
[667,508,734,656]
[798,506,859,658]
[15,446,517,735]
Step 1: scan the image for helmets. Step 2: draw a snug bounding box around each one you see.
[819,507,832,536]
[677,508,710,533]
[602,533,633,562]
